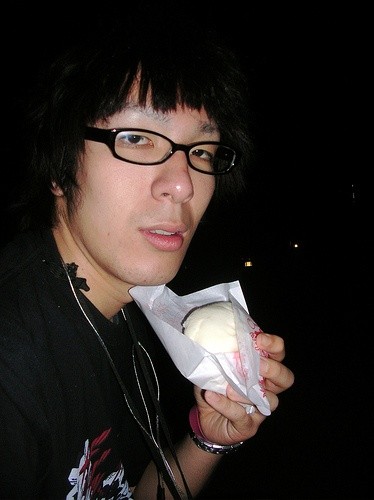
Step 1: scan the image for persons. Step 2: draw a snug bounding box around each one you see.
[0,25,295,500]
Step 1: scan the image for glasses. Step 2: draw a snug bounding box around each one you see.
[78,125,236,176]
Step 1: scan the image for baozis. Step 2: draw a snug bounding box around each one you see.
[182,299,265,383]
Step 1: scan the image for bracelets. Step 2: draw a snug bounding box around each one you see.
[188,405,244,455]
